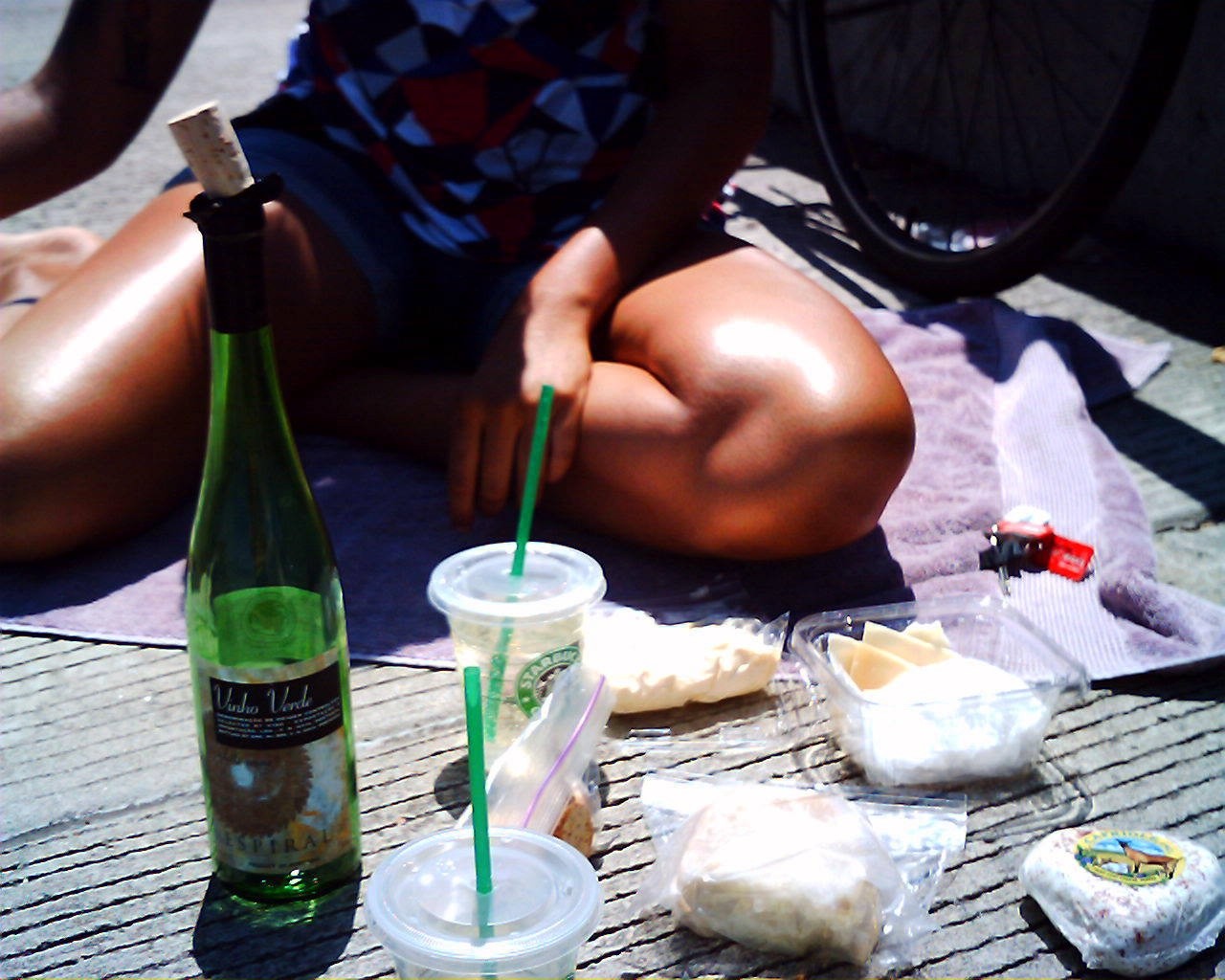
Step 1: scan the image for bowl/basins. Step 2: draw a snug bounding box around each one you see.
[786,593,1092,800]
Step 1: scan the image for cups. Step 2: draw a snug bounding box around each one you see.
[428,541,607,781]
[364,826,601,980]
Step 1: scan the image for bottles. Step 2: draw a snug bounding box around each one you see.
[166,100,361,913]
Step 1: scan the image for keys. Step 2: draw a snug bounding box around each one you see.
[987,504,1053,596]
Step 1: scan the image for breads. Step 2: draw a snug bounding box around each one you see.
[669,778,882,965]
[1018,829,1224,977]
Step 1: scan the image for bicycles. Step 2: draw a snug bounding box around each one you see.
[779,0,1204,300]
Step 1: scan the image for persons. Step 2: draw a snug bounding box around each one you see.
[0,0,916,605]
